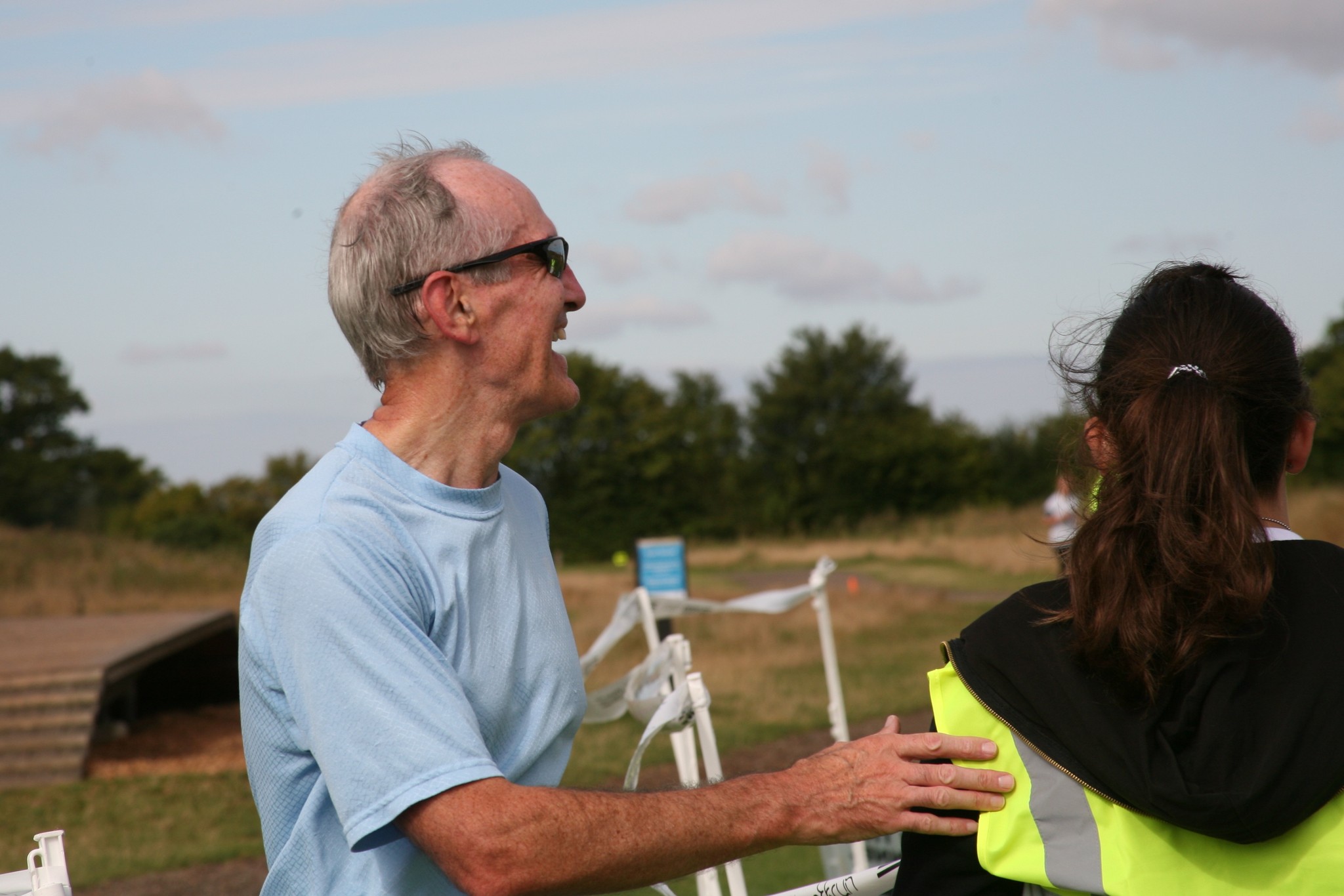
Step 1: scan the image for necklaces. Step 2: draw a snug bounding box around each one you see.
[1262,517,1292,530]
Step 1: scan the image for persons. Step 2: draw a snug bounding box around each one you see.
[1041,470,1084,576]
[239,139,1017,896]
[895,260,1344,896]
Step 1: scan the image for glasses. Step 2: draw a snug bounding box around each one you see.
[390,237,569,296]
